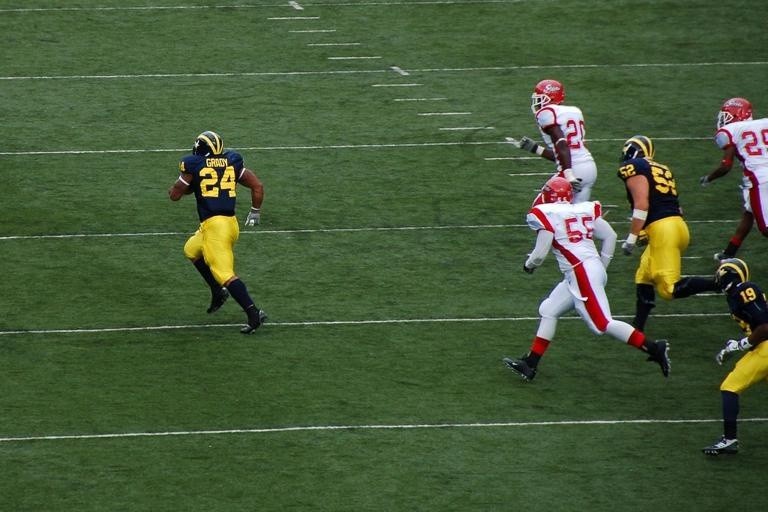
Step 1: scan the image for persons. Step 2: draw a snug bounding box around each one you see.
[618,134,722,331]
[701,257,768,456]
[501,176,672,384]
[166,128,271,337]
[501,77,599,211]
[699,96,767,265]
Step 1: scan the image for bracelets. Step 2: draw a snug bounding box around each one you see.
[535,144,546,158]
[625,232,639,245]
[740,336,753,352]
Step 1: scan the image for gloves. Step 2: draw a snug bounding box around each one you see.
[725,339,739,355]
[621,241,635,256]
[715,349,729,366]
[568,177,583,193]
[699,175,707,188]
[523,264,535,274]
[519,136,539,154]
[244,207,261,228]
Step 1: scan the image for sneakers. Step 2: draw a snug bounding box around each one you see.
[206,287,230,314]
[702,435,739,455]
[240,309,268,335]
[502,354,537,383]
[645,338,672,378]
[713,250,736,262]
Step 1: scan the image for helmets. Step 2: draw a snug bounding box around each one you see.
[716,97,753,131]
[714,257,749,296]
[530,79,564,114]
[621,134,655,161]
[541,176,574,204]
[192,130,224,157]
